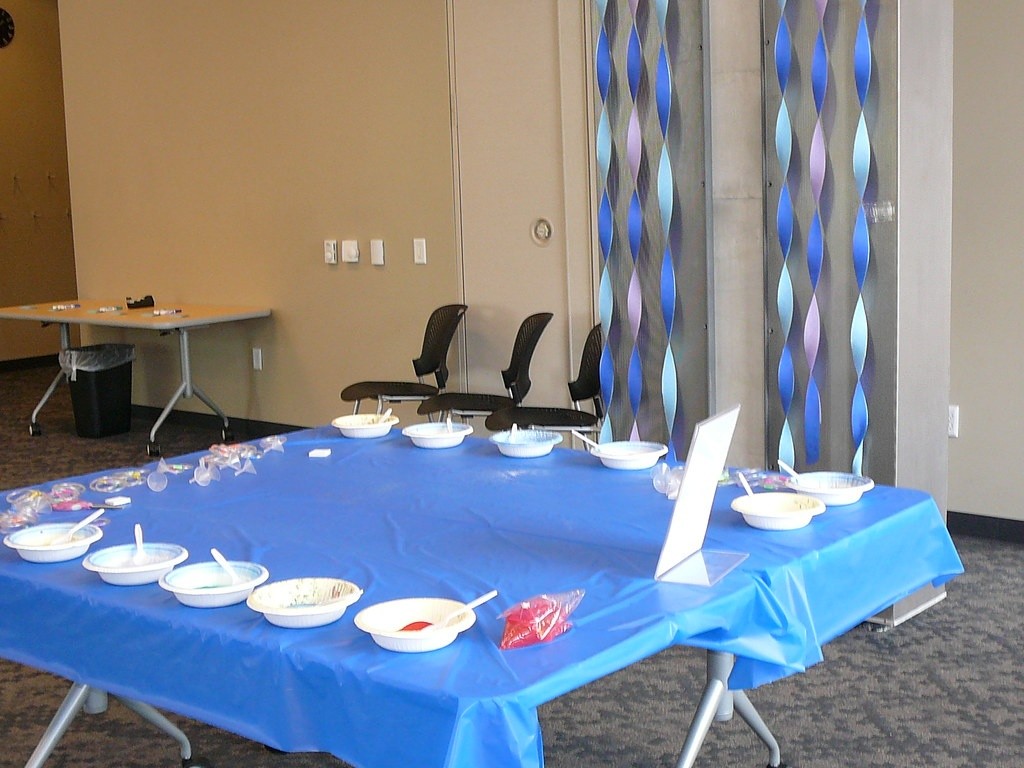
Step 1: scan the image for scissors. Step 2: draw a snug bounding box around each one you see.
[52,500,123,511]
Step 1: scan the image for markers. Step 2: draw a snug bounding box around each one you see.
[53,304,80,310]
[98,306,123,312]
[154,309,182,315]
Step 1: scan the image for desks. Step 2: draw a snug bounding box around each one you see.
[0,418,968,768]
[0,299,272,455]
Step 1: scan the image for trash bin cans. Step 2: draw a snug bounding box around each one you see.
[58,343,136,438]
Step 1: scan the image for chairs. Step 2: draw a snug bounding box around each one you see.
[338,304,469,416]
[416,311,555,422]
[483,323,604,451]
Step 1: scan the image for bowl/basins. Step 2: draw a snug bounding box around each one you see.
[3,521,104,564]
[82,541,189,586]
[590,441,669,471]
[401,421,473,449]
[246,576,361,629]
[354,597,476,654]
[158,560,269,609]
[489,430,564,458]
[730,491,826,531]
[783,471,874,507]
[331,413,400,439]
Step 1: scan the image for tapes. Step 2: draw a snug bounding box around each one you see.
[130,297,145,303]
